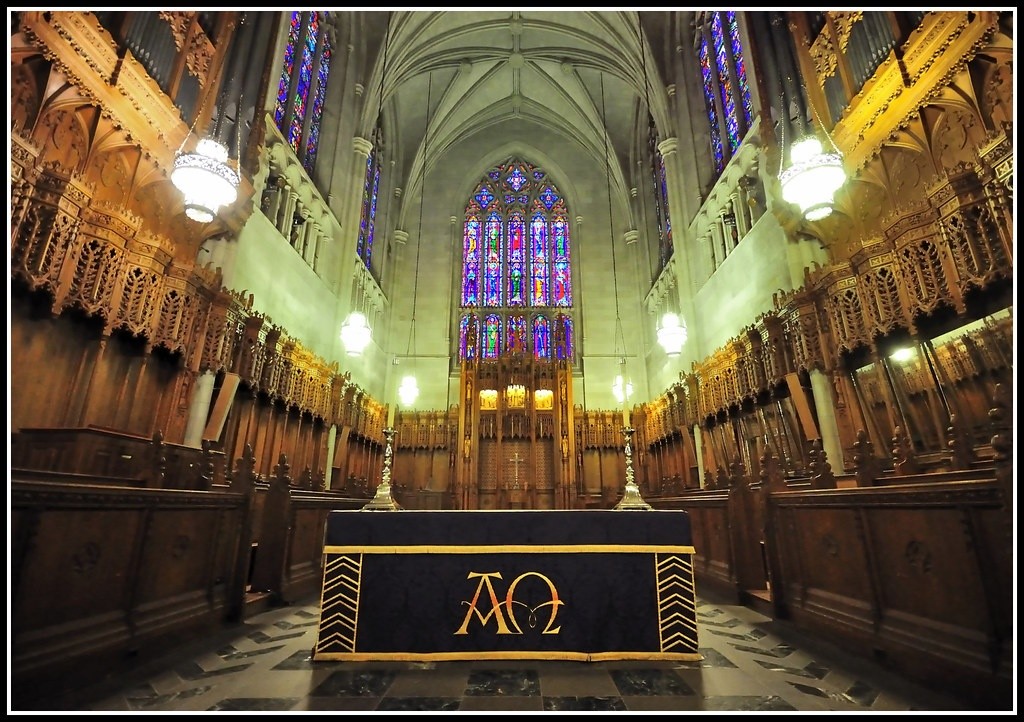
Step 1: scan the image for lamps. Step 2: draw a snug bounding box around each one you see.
[635,12,688,362]
[337,10,392,358]
[769,10,846,224]
[171,11,249,225]
[598,72,634,401]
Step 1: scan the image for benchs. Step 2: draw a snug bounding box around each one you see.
[645,382,1013,711]
[13,418,407,711]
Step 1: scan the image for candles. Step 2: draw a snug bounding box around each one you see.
[388,358,400,429]
[619,359,630,428]
